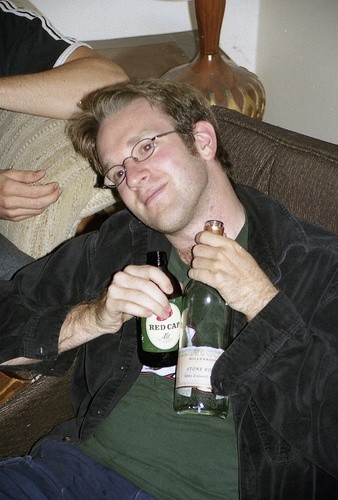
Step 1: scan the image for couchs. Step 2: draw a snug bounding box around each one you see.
[0,105,338,460]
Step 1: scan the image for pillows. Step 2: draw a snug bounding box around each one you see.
[0,109,119,260]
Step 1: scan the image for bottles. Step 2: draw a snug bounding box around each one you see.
[174,220,233,420]
[136,250,183,367]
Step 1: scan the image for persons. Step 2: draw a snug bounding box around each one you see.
[0,77,338,500]
[0,0,131,223]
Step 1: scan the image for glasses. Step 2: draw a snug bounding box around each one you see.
[102,127,188,188]
